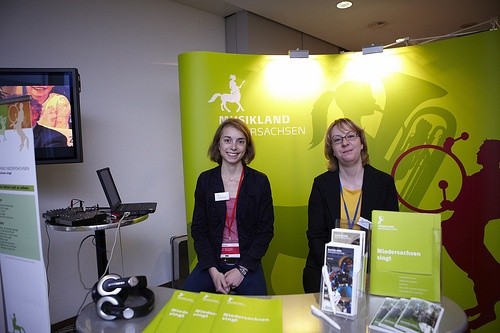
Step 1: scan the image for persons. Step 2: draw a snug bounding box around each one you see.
[304,118,399,293]
[181,119,274,295]
[25,86,71,147]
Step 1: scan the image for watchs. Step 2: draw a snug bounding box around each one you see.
[237,266,248,276]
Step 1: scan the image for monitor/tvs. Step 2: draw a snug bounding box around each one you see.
[0,67,82,165]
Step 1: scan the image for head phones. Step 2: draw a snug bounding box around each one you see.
[91,276,148,303]
[95,287,154,321]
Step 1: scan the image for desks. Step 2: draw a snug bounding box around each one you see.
[46,208,149,279]
[74,284,469,333]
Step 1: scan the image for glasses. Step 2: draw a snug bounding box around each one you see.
[331,129,359,144]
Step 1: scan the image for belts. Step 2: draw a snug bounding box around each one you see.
[219,258,241,265]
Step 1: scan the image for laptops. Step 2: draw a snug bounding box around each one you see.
[96,167,157,214]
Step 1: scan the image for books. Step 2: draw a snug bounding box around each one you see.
[369,296,444,333]
[141,290,283,333]
[369,209,442,302]
